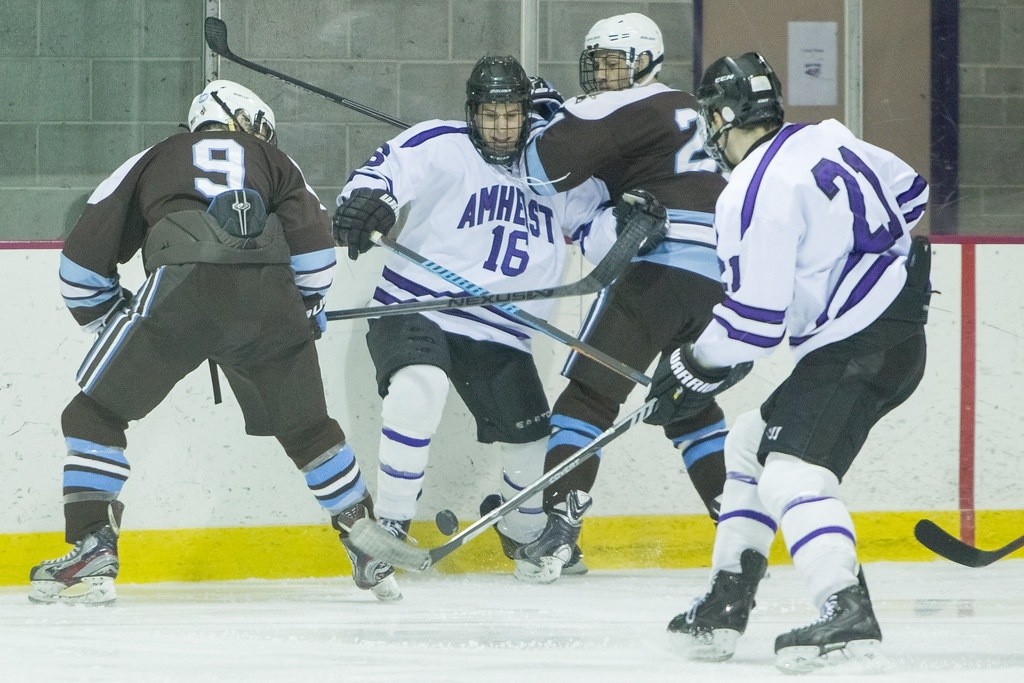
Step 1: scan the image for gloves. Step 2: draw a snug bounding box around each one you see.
[527,76,564,117]
[613,190,668,257]
[305,295,327,341]
[330,187,398,261]
[644,343,729,427]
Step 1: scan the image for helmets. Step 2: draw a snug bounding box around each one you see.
[465,54,531,120]
[698,52,784,130]
[186,80,277,137]
[584,12,665,86]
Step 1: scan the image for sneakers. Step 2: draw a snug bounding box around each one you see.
[28,526,119,608]
[338,528,404,604]
[773,566,882,672]
[666,550,768,660]
[512,492,592,585]
[376,517,410,545]
[492,515,589,575]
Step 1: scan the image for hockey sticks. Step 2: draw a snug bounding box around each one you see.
[323,212,653,321]
[369,229,654,389]
[346,396,660,575]
[913,518,1024,569]
[203,16,413,130]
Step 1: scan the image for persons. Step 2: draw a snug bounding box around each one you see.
[26,80,402,604]
[337,11,732,589]
[644,52,930,673]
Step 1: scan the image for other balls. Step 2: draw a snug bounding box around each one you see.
[434,510,458,536]
